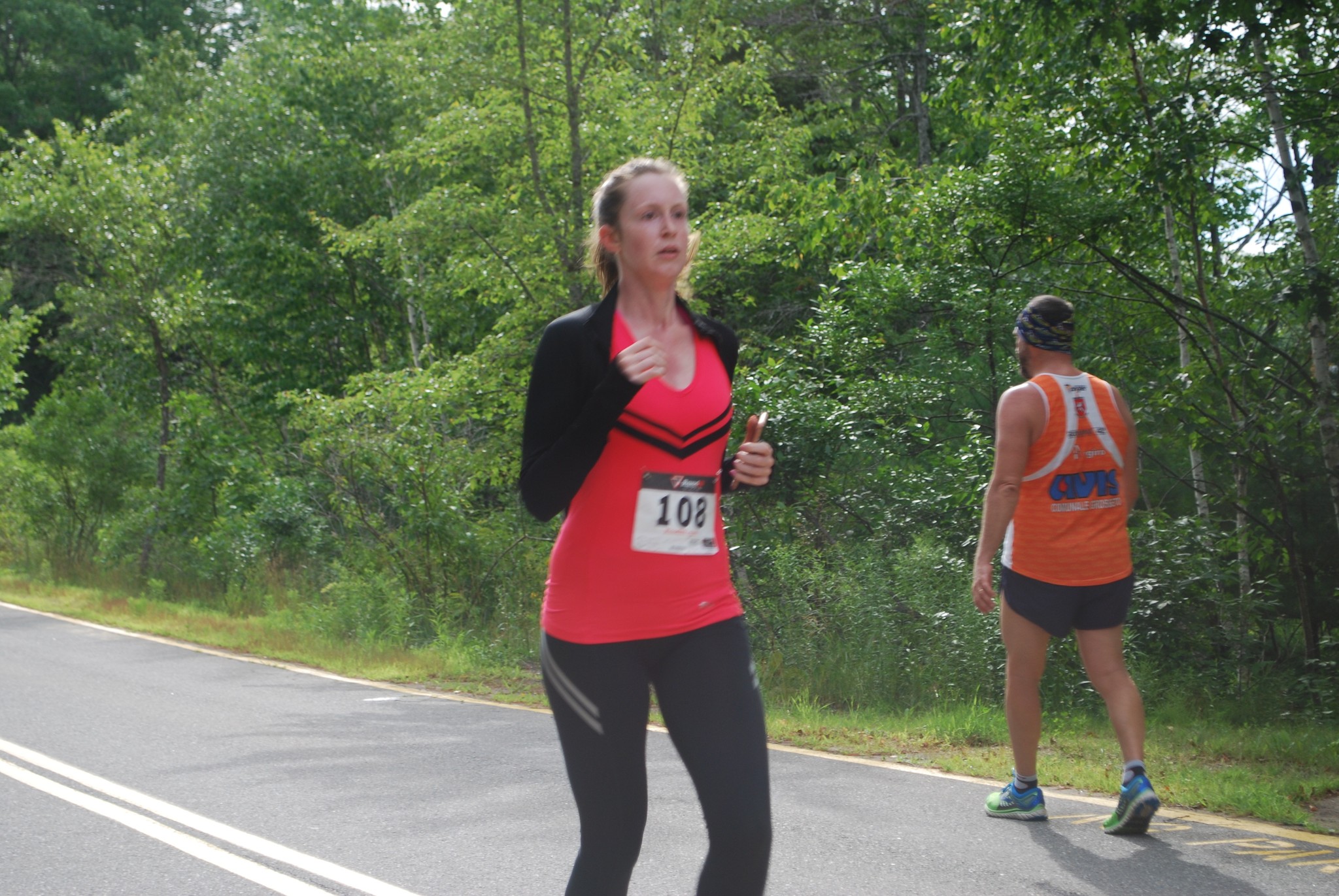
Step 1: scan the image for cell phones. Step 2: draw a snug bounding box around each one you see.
[730,412,768,491]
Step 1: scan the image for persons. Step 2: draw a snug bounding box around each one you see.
[971,295,1160,835]
[518,159,773,896]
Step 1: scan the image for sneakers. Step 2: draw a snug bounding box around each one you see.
[1103,775,1159,835]
[985,779,1047,821]
